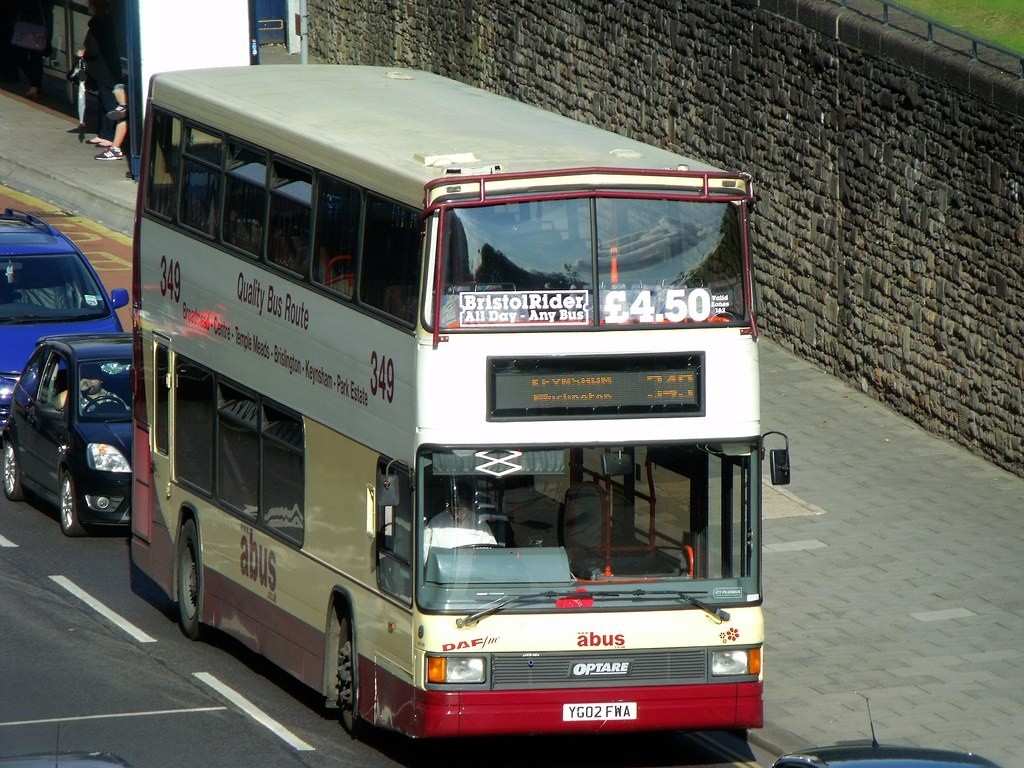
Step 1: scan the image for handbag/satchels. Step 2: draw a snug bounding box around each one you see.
[66,56,89,86]
[11,21,47,50]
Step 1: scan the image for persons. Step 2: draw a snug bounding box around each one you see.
[10,0,51,98]
[95,83,128,161]
[418,487,498,567]
[48,361,133,414]
[77,0,123,150]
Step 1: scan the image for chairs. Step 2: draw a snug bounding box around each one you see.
[324,250,722,326]
[560,480,610,575]
[11,257,69,312]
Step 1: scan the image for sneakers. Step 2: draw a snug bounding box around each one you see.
[106,103,128,120]
[95,148,122,160]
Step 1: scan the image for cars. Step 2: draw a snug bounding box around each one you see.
[771,691,1007,768]
[3,332,133,539]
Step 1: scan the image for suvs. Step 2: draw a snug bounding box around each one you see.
[0,207,130,446]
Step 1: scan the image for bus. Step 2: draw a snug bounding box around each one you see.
[127,69,791,740]
